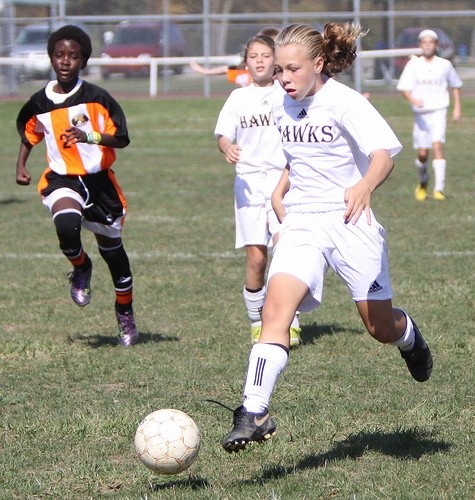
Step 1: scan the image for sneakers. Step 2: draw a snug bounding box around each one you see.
[68,254,93,307]
[222,405,276,452]
[415,182,428,201]
[289,327,302,345]
[432,190,445,200]
[112,302,139,347]
[250,323,263,340]
[395,308,433,383]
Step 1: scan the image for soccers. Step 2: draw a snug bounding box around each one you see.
[134,409,201,474]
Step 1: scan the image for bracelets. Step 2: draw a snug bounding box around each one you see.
[87,131,101,144]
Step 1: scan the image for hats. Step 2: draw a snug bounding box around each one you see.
[417,30,437,40]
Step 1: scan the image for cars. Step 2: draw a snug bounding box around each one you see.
[99,20,187,79]
[395,27,457,78]
[6,23,90,82]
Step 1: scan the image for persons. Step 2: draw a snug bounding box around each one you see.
[236,27,371,98]
[396,29,463,202]
[222,21,433,453]
[214,35,302,348]
[16,25,138,347]
